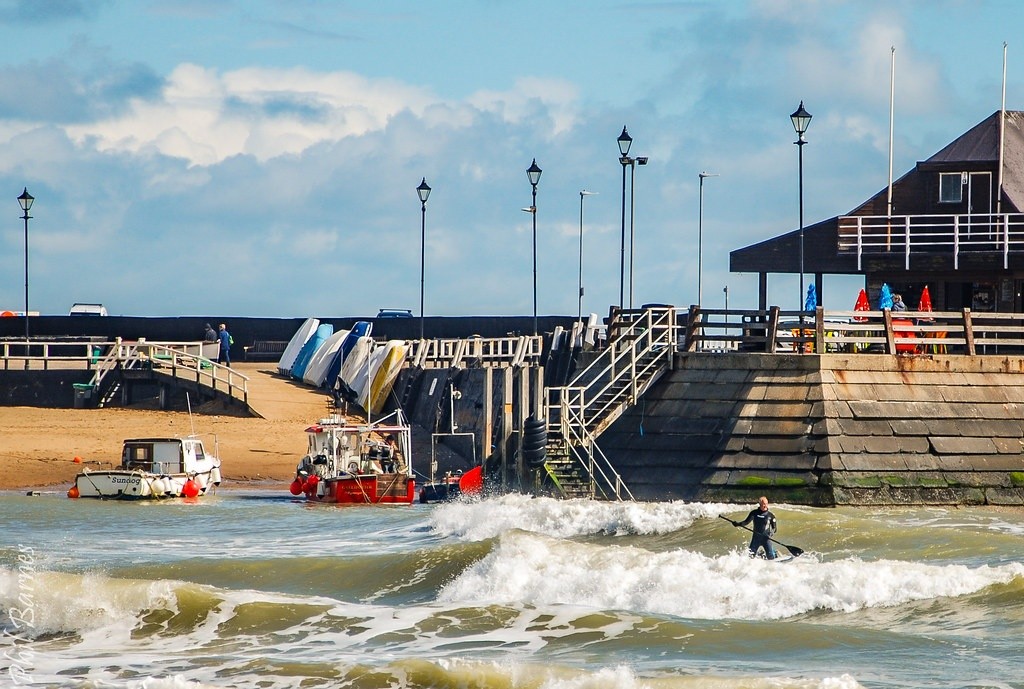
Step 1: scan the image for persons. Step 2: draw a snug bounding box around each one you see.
[218,323,230,368]
[732,496,777,560]
[204,323,217,341]
[381,434,399,473]
[891,295,908,320]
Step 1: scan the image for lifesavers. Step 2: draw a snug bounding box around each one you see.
[523,416,547,467]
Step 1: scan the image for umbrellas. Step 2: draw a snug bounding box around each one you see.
[853,289,870,321]
[805,284,817,317]
[878,282,894,310]
[917,285,934,323]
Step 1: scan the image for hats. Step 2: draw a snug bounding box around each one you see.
[387,434,395,440]
[219,324,227,331]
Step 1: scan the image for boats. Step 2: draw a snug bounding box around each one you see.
[276,317,322,378]
[419,381,485,502]
[300,319,409,417]
[283,343,417,504]
[67,391,222,504]
[289,323,333,382]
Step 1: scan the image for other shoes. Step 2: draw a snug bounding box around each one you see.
[226,363,232,369]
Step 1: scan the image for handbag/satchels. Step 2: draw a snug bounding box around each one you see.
[228,336,235,347]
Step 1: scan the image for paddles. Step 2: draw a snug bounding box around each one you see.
[718,514,805,557]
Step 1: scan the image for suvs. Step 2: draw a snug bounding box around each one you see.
[376,308,413,320]
[69,303,107,316]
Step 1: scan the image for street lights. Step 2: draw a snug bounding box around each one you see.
[516,158,542,336]
[17,186,35,373]
[695,171,720,353]
[614,123,633,362]
[789,99,814,359]
[415,177,432,343]
[577,190,599,324]
